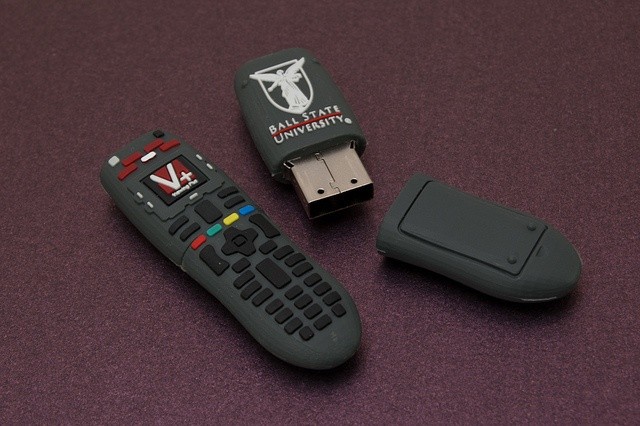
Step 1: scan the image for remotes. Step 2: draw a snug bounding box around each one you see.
[99,122,366,372]
